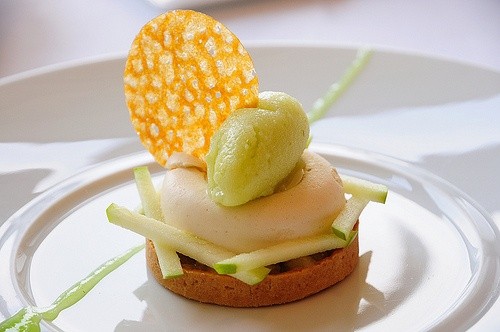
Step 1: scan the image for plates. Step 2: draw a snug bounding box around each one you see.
[0,142,500,332]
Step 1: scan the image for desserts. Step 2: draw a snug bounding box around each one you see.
[107,9,391,306]
